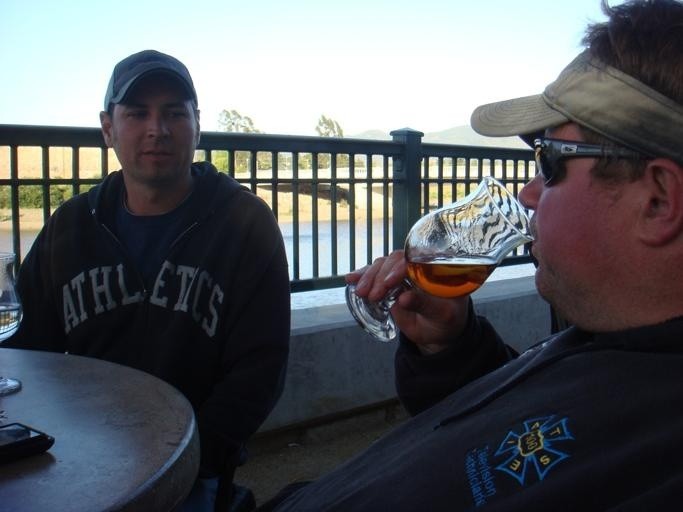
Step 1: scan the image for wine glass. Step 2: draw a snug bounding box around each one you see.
[344,176,535,343]
[0,253,25,394]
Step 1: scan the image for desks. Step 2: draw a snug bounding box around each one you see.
[0,344,201,511]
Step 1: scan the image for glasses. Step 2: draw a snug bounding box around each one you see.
[533,137,650,188]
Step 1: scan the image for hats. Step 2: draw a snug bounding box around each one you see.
[471,48,683,163]
[104,49,196,112]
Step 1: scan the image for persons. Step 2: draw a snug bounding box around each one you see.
[272,0,683,512]
[13,50,291,512]
